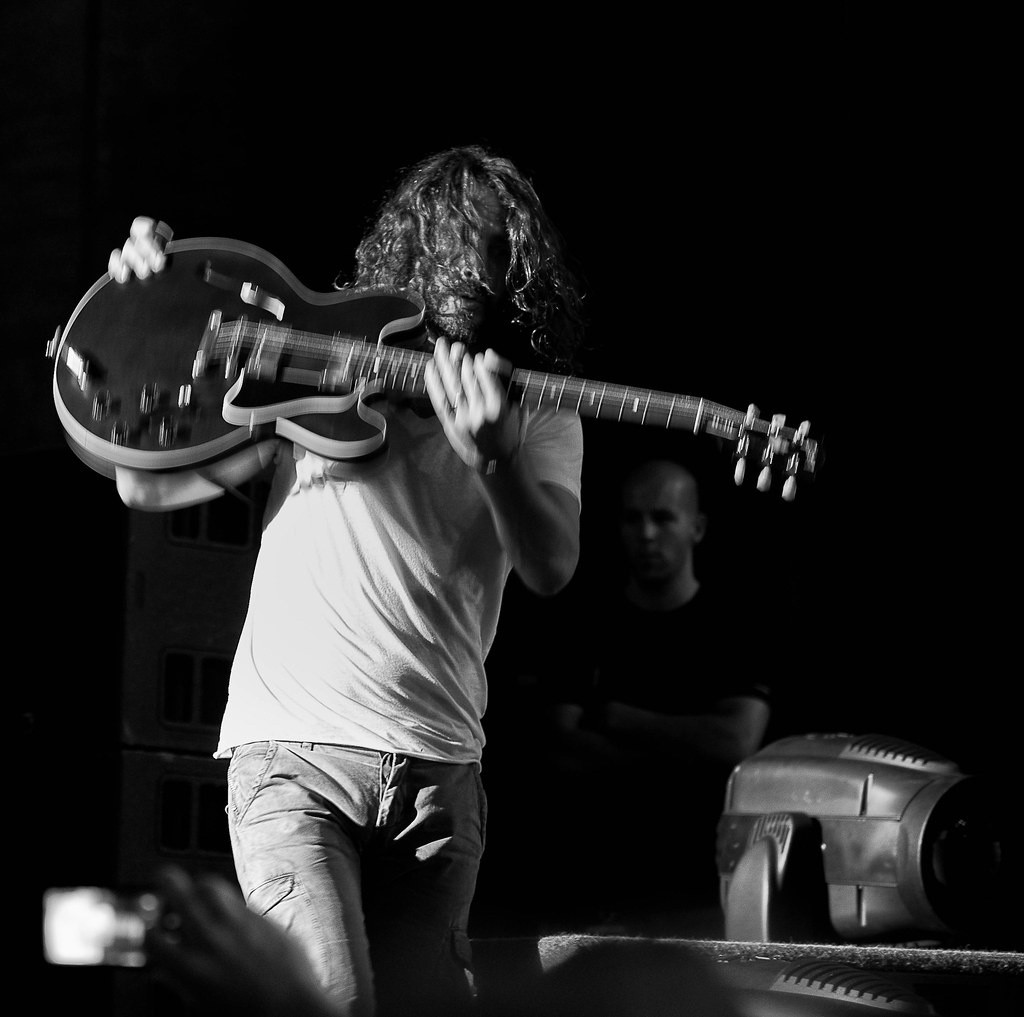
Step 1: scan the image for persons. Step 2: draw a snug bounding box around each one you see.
[89,144,586,1011]
[557,458,777,937]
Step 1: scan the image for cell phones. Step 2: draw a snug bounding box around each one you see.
[44,885,180,969]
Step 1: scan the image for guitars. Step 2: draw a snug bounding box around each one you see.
[47,235,822,502]
